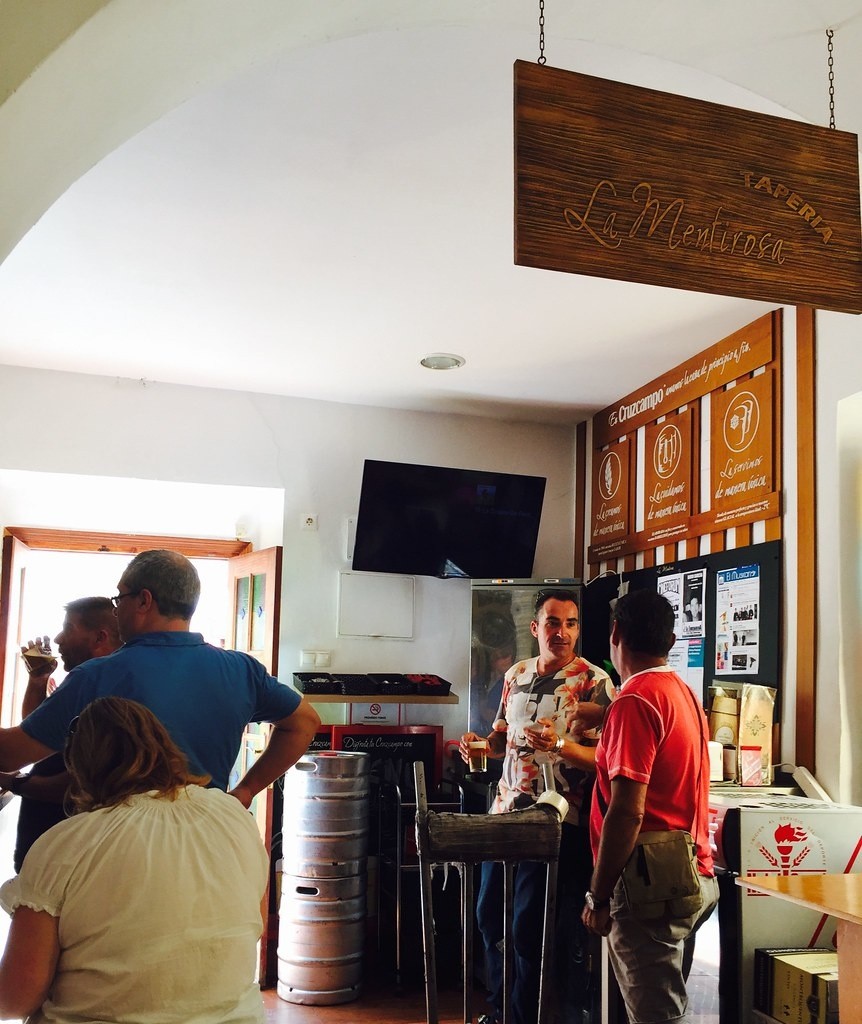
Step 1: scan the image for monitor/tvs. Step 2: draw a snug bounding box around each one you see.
[352,459,547,580]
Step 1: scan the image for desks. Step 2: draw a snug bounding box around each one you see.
[737,875,862,1024]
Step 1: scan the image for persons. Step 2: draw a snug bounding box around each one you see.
[0,550,321,876]
[460,591,619,1024]
[0,697,268,1024]
[469,647,514,736]
[568,589,720,1024]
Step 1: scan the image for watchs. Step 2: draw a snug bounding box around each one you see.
[585,891,610,911]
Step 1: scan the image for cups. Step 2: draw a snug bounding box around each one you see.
[741,745,762,786]
[20,638,63,672]
[708,740,736,782]
[468,740,488,772]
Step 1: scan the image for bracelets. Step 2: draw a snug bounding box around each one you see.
[553,736,564,753]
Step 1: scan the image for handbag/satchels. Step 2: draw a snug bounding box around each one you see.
[621,831,704,919]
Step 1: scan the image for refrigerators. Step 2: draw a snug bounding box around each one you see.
[704,793,862,1024]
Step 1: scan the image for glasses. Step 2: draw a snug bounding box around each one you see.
[66,715,81,738]
[111,591,158,608]
[536,588,576,601]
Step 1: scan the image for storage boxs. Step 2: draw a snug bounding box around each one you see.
[754,947,838,1024]
[704,696,779,782]
[292,671,453,696]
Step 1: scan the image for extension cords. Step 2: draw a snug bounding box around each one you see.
[792,766,833,803]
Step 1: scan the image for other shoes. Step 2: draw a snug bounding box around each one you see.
[477,1014,509,1024]
